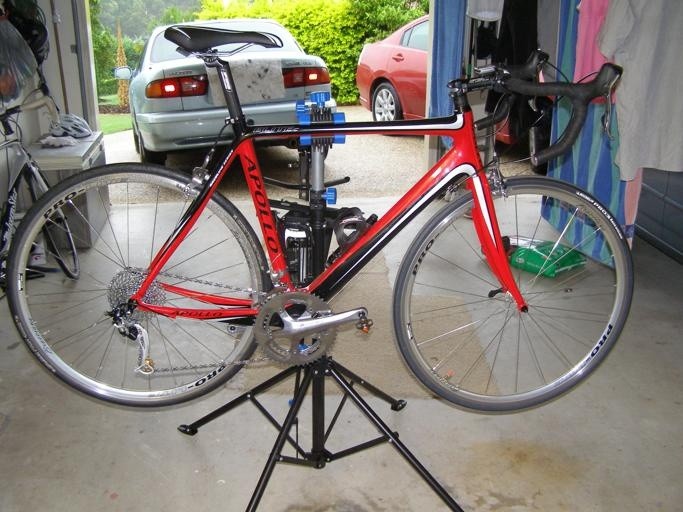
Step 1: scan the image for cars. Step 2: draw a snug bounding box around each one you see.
[114,19,336,165]
[355,15,555,144]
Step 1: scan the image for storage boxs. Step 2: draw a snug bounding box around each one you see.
[18,132,110,250]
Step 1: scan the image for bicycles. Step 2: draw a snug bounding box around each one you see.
[0,96,80,299]
[5,25,632,412]
